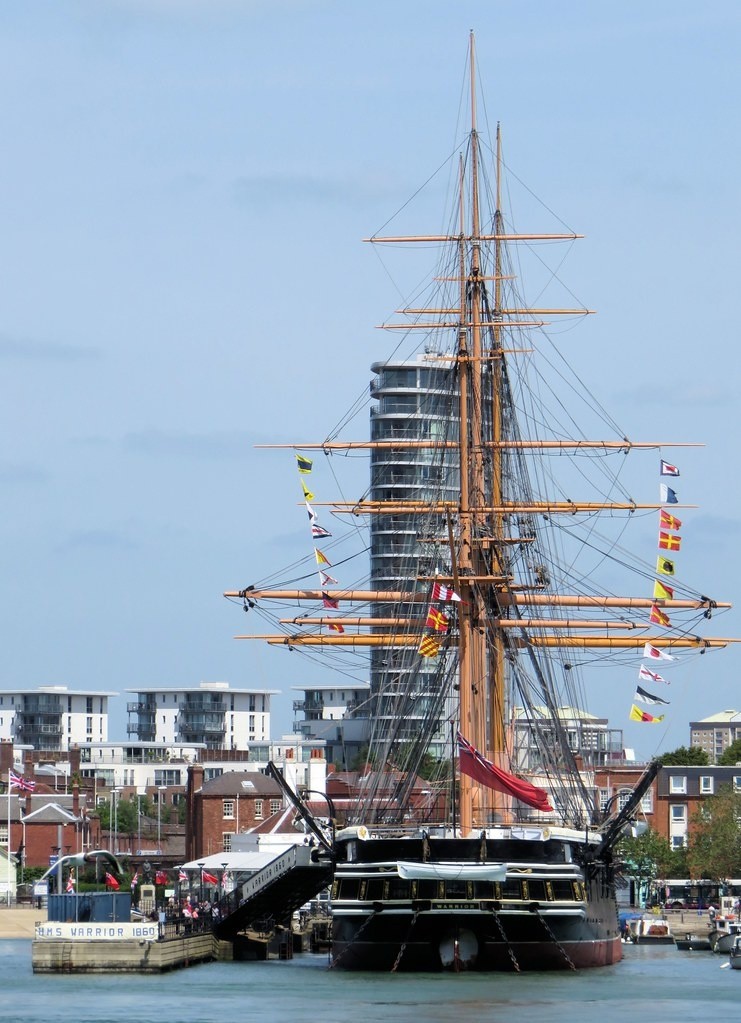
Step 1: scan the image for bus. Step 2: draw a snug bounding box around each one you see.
[649,879,741,913]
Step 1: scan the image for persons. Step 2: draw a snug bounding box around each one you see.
[708,902,720,928]
[142,858,151,884]
[732,901,739,914]
[697,896,702,916]
[150,900,219,933]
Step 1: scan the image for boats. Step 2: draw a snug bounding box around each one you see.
[210,28,741,973]
[631,915,674,946]
[676,918,741,971]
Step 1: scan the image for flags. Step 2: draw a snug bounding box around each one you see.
[178,870,188,882]
[215,873,226,891]
[456,731,554,812]
[631,459,681,723]
[419,635,440,658]
[425,607,449,631]
[106,872,119,890]
[66,872,74,894]
[156,871,170,884]
[10,771,35,791]
[131,873,138,889]
[294,454,344,633]
[202,870,218,884]
[433,583,468,606]
